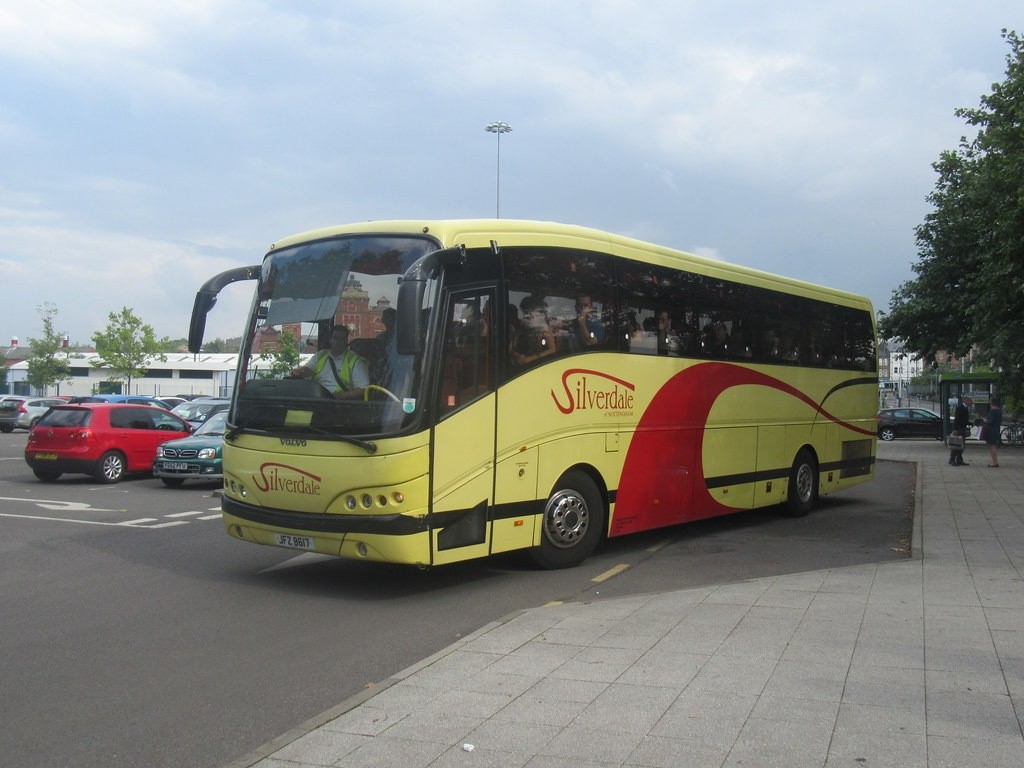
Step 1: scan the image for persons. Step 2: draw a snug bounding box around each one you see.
[975,395,1002,467]
[376,293,858,373]
[948,396,973,466]
[290,325,371,399]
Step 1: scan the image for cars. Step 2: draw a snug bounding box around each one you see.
[0,394,231,433]
[152,409,230,490]
[878,408,954,441]
[24,402,196,484]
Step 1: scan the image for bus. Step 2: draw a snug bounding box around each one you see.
[187,219,881,576]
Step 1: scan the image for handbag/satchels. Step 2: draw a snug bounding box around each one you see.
[947,432,964,450]
[975,426,988,441]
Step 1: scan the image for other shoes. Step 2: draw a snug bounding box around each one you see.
[948,459,958,466]
[987,462,999,467]
[958,462,969,466]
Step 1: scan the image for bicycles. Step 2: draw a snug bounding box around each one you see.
[1000,419,1024,446]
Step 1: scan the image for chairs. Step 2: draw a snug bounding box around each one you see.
[547,317,580,355]
[349,338,388,380]
